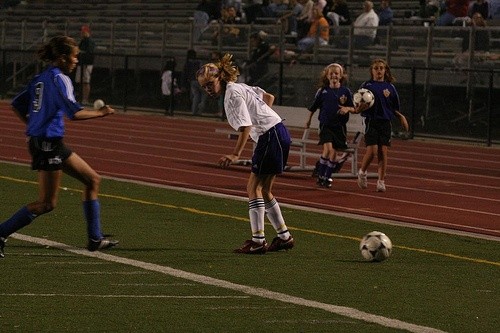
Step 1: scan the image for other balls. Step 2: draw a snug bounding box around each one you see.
[94,100,104,110]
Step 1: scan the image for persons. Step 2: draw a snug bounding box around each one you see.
[194,18,220,46]
[425,0,500,27]
[197,0,290,42]
[185,50,207,116]
[247,34,269,86]
[355,59,409,192]
[352,0,379,48]
[276,0,349,37]
[297,6,330,51]
[195,54,295,255]
[377,0,393,26]
[0,37,119,257]
[304,63,359,188]
[462,12,490,52]
[74,26,97,105]
[161,60,181,116]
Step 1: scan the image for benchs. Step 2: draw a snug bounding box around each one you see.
[0,0,500,73]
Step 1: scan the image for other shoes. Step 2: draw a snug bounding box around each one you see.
[377,179,385,192]
[316,175,334,188]
[81,101,88,105]
[358,168,368,189]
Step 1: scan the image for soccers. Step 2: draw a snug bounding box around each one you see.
[360,230,392,262]
[353,88,374,108]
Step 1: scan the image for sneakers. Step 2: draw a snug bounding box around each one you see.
[235,240,268,254]
[0,235,6,258]
[87,236,121,252]
[267,236,295,252]
